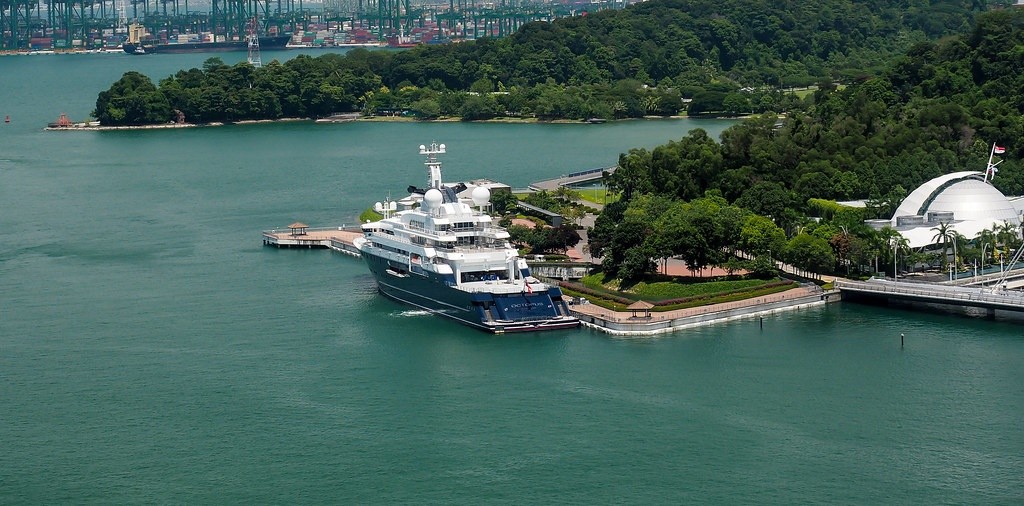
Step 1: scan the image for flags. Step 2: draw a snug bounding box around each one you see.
[992,142,1005,153]
[524,278,532,294]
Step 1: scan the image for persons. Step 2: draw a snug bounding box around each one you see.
[272,225,281,233]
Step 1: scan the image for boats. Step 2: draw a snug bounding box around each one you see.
[353,138,582,334]
[123,36,293,56]
